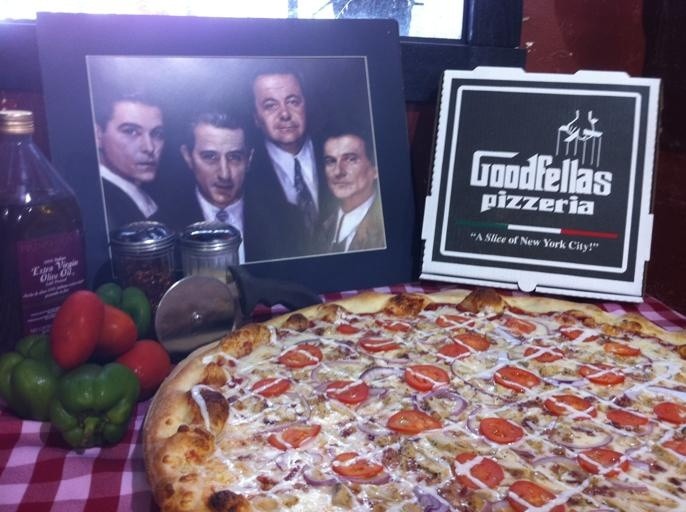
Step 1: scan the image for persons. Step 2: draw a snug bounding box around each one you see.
[317,122,385,250]
[96,82,175,237]
[245,61,337,260]
[157,109,267,263]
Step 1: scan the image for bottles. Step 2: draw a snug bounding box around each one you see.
[1,107,90,359]
[107,216,243,325]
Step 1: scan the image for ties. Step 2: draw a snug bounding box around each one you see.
[294,159,318,241]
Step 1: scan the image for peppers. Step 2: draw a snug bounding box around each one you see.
[0,280,176,451]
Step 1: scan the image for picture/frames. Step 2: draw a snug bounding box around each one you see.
[36,13,417,296]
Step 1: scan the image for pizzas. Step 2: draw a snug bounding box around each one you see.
[142,286,685,512]
